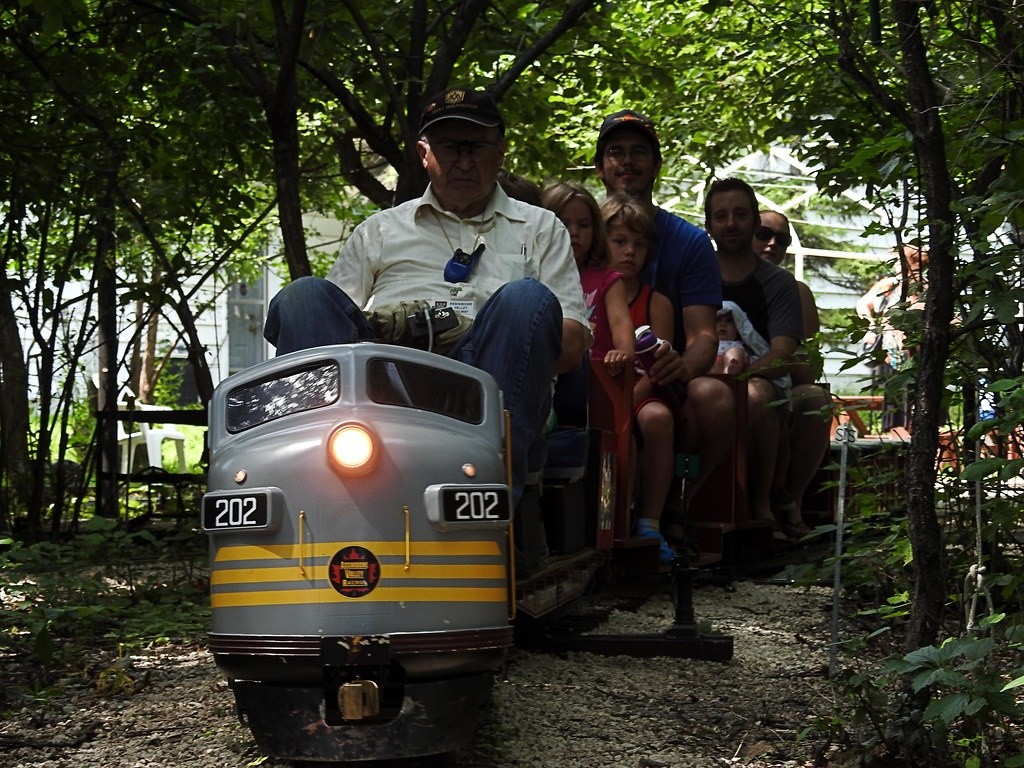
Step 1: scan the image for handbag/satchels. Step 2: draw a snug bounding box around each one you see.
[360,298,473,352]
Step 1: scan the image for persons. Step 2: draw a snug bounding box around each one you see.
[978,368,1005,446]
[504,109,833,566]
[261,90,592,578]
[856,244,929,434]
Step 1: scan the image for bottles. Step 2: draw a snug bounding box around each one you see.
[634,325,679,400]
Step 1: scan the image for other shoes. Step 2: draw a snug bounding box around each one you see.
[785,519,813,539]
[640,530,676,564]
[687,540,699,561]
[761,518,787,542]
[664,534,689,568]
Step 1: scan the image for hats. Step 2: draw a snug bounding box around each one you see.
[595,110,660,148]
[419,89,502,136]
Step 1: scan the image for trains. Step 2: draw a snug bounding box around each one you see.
[195,315,1024,768]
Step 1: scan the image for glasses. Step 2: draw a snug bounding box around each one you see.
[755,226,792,248]
[605,145,650,162]
[420,140,498,161]
